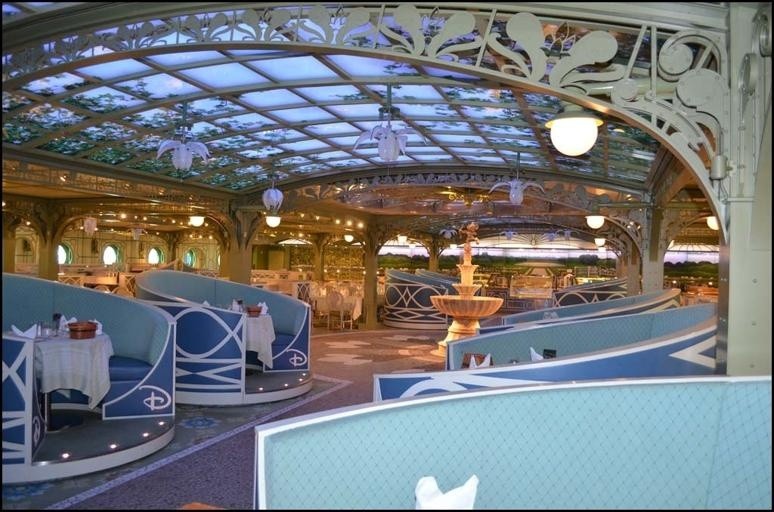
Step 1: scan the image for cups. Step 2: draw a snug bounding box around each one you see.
[41,328,49,337]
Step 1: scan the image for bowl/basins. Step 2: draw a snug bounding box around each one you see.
[67,322,96,339]
[247,306,262,317]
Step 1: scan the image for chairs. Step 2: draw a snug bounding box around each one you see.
[67,259,721,330]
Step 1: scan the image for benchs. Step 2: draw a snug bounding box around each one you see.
[2,270,177,460]
[137,267,311,390]
[243,289,774,512]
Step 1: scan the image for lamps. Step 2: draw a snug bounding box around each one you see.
[156,100,210,171]
[488,152,545,206]
[545,70,605,156]
[262,173,284,211]
[435,222,560,243]
[585,200,605,230]
[350,83,427,163]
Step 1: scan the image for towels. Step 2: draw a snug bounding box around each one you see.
[9,315,104,339]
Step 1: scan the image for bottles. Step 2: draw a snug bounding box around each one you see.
[36,321,42,337]
